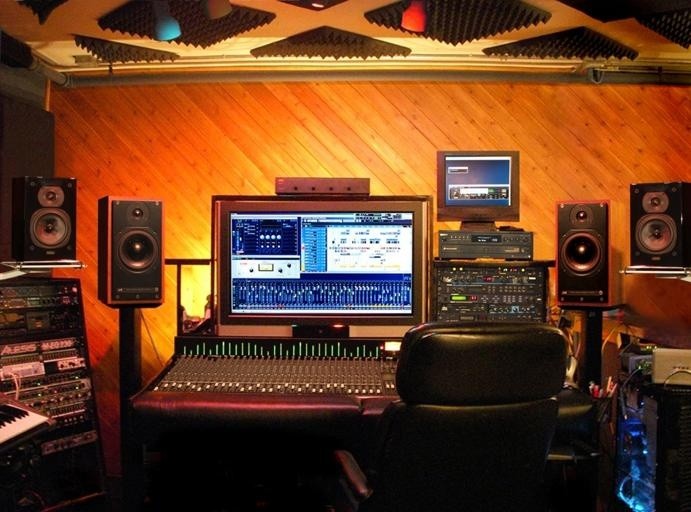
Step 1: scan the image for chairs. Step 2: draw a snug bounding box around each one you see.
[328,318,607,512]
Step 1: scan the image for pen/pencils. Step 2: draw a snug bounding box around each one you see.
[588,375,618,423]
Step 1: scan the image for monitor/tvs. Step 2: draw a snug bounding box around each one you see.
[210,195,433,326]
[437,151,519,221]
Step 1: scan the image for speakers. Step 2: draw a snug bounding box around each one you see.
[98,196,166,308]
[555,200,623,308]
[12,175,77,261]
[630,181,691,267]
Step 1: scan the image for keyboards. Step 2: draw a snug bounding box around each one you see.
[147,338,402,396]
